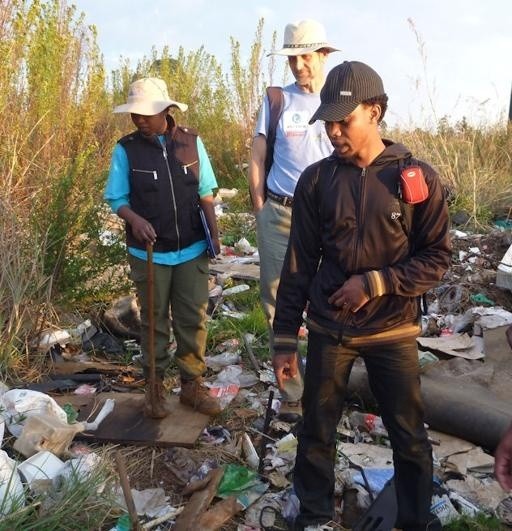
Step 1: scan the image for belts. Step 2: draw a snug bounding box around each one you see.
[265,187,293,207]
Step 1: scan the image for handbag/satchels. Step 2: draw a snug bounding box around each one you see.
[400,167,429,204]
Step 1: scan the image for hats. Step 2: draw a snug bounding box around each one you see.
[265,20,342,57]
[112,78,188,116]
[308,60,385,125]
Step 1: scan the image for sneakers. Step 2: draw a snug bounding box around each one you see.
[279,400,303,422]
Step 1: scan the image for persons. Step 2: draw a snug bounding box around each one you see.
[493,428,512,494]
[104,77,221,419]
[271,60,453,531]
[247,20,343,422]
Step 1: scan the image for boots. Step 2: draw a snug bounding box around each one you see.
[144,376,167,418]
[180,375,221,415]
